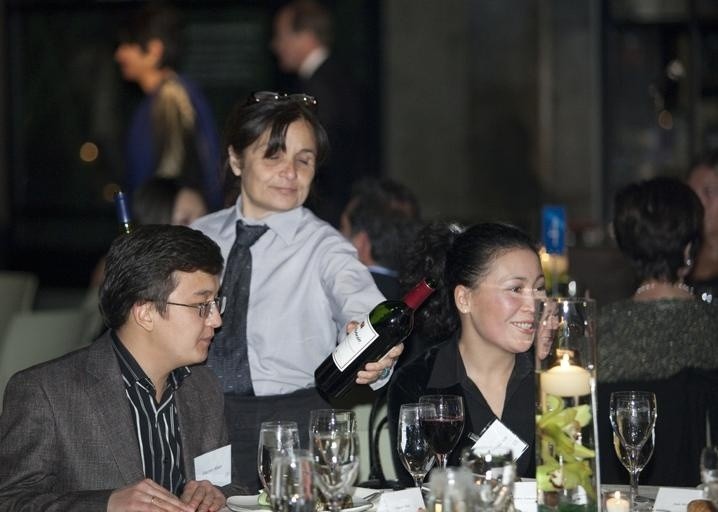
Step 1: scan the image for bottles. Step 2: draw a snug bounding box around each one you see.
[113,186,142,236]
[534,298,601,512]
[313,274,438,400]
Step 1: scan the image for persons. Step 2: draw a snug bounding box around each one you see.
[188,90,404,494]
[583,175,718,487]
[387,221,547,486]
[270,3,385,225]
[624,156,718,312]
[112,17,226,224]
[0,224,248,512]
[339,179,421,300]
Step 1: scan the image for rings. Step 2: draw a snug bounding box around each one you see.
[151,496,155,504]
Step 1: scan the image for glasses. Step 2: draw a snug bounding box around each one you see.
[146,295,227,318]
[239,90,320,111]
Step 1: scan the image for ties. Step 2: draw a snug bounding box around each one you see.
[204,219,270,398]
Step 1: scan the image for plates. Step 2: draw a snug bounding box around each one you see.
[226,490,374,512]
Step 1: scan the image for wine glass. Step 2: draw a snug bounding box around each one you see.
[257,409,361,512]
[397,394,517,512]
[609,391,658,511]
